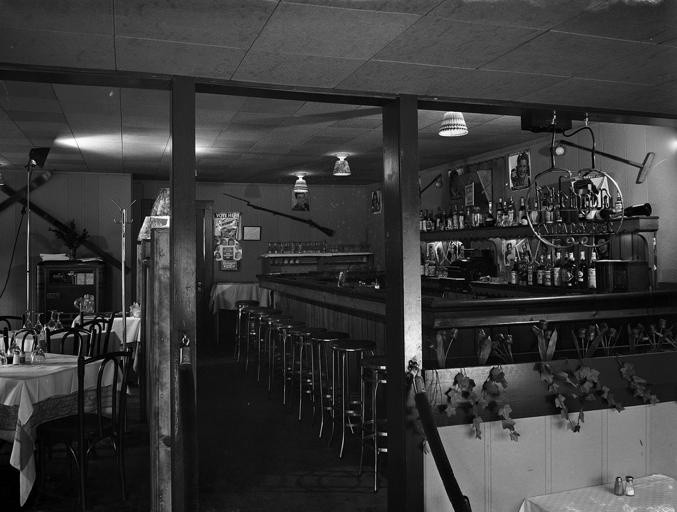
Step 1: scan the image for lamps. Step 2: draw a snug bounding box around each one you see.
[437,112,467,137]
[292,173,307,192]
[332,153,351,176]
[25,147,51,310]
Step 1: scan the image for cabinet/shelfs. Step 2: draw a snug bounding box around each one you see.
[257,253,374,274]
[35,263,113,319]
[421,217,658,296]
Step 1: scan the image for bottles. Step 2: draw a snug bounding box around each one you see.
[336,271,344,288]
[624,474,634,495]
[506,250,596,289]
[614,476,624,495]
[419,205,482,231]
[611,203,651,219]
[6,345,25,365]
[614,192,622,214]
[484,185,562,226]
[420,260,435,277]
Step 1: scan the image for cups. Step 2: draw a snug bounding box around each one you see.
[29,345,46,365]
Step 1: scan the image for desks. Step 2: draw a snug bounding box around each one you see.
[517,473,676,511]
[210,282,257,345]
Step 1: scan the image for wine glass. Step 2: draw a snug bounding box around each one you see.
[22,309,64,334]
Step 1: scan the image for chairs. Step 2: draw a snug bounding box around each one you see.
[0,304,142,511]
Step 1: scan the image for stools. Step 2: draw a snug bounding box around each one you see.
[234,299,394,494]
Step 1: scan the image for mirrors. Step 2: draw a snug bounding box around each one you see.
[449,159,492,217]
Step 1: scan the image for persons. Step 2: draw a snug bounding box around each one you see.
[509,152,530,191]
[428,246,436,261]
[214,226,241,261]
[505,241,515,265]
[292,192,309,211]
[437,244,452,266]
[522,244,531,263]
[372,192,380,213]
